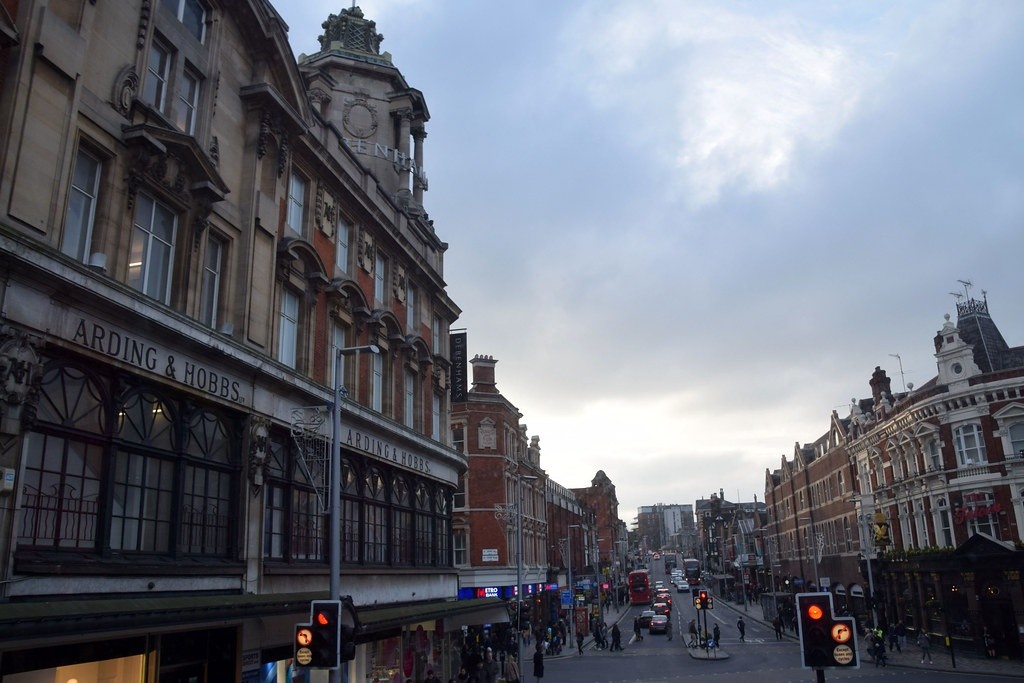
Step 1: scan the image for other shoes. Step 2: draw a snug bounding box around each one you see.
[921,660,924,663]
[593,645,625,652]
[930,660,933,665]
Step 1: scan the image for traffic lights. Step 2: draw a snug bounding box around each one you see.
[292,599,342,670]
[694,590,714,609]
[340,623,355,663]
[795,592,862,670]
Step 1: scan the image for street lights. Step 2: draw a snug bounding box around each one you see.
[716,536,728,602]
[759,528,778,623]
[567,523,581,650]
[732,533,747,612]
[596,539,605,623]
[846,498,878,629]
[518,472,539,683]
[799,516,819,591]
[328,344,380,683]
[613,541,620,613]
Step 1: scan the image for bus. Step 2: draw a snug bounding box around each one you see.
[683,558,701,586]
[628,568,654,605]
[665,553,677,574]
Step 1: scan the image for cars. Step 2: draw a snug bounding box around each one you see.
[638,611,656,628]
[649,614,670,634]
[648,549,665,560]
[670,567,684,587]
[653,580,673,611]
[677,581,689,594]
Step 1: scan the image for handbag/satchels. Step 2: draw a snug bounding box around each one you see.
[515,678,520,683]
[541,641,549,649]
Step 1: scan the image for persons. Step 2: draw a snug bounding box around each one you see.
[737,616,745,642]
[633,619,644,641]
[710,567,717,575]
[576,613,623,655]
[834,607,933,666]
[424,610,567,683]
[665,619,672,641]
[773,603,799,640]
[747,585,769,606]
[600,590,625,613]
[982,627,995,659]
[687,619,720,647]
